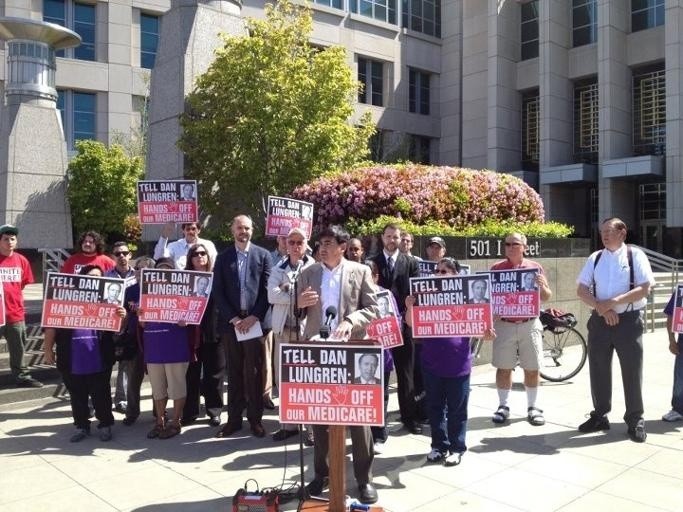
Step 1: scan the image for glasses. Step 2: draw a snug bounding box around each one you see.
[113,251,129,257]
[287,239,303,246]
[504,242,525,247]
[191,251,206,257]
[434,269,454,274]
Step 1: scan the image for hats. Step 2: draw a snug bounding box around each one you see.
[154,257,175,270]
[1,224,18,236]
[426,236,447,252]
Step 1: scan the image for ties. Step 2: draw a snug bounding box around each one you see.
[385,257,396,277]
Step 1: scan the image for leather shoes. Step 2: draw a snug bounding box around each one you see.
[303,431,314,446]
[250,422,264,436]
[357,482,377,504]
[263,398,274,411]
[403,420,422,433]
[210,416,221,426]
[304,477,330,496]
[216,424,241,438]
[271,429,298,441]
[179,417,195,426]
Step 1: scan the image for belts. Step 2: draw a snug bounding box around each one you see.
[284,325,300,332]
[500,317,534,324]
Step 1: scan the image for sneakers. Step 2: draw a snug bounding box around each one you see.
[69,427,90,443]
[88,403,138,426]
[146,422,163,439]
[426,447,447,462]
[14,376,43,388]
[444,449,464,465]
[99,425,111,440]
[627,425,646,443]
[157,422,180,440]
[661,408,683,423]
[578,416,610,433]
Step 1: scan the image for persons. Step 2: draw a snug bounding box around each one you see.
[0,224,42,388]
[662,286,682,421]
[180,183,194,202]
[575,216,655,441]
[488,231,552,424]
[520,272,538,292]
[302,205,311,220]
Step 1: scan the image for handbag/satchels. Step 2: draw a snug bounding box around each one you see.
[112,332,138,361]
[539,307,577,335]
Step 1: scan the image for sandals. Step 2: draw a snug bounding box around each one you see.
[527,406,544,426]
[491,404,510,423]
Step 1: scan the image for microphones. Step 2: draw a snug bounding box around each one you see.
[324,306,337,326]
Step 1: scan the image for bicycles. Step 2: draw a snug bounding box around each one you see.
[466,298,587,385]
